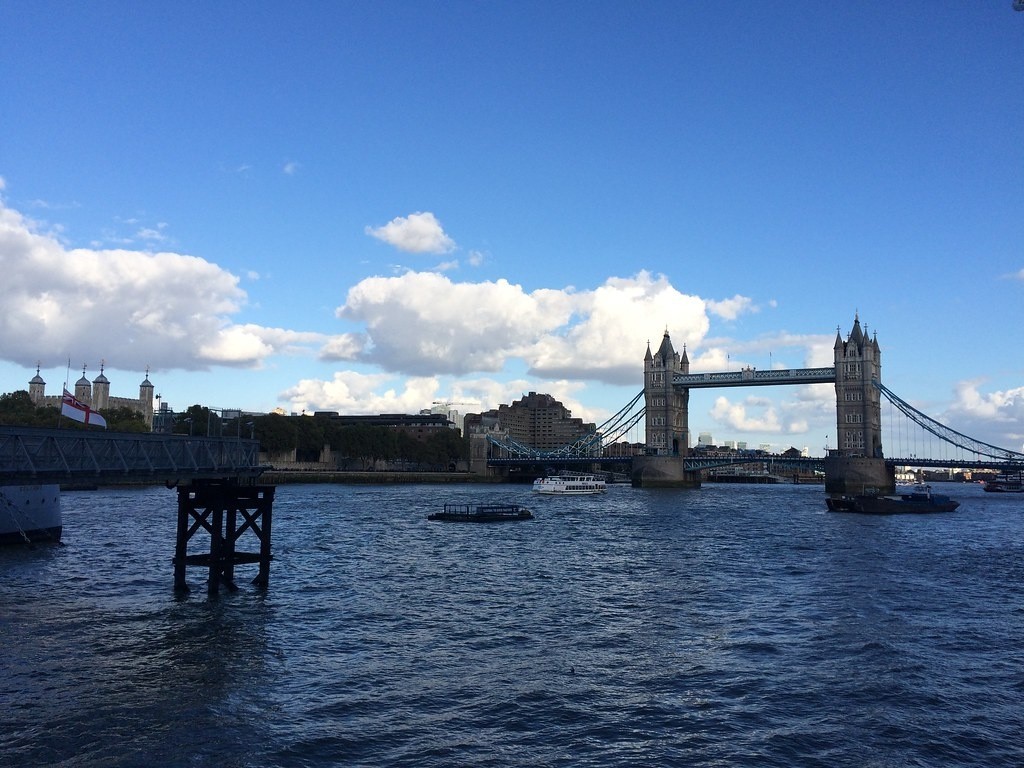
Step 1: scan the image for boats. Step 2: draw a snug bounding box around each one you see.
[895,480,898,484]
[896,481,911,486]
[613,481,632,487]
[532,475,608,495]
[920,479,926,485]
[983,481,1024,492]
[973,480,980,483]
[914,481,919,484]
[963,481,966,484]
[993,474,1024,484]
[428,501,536,522]
[860,493,960,515]
[980,480,984,484]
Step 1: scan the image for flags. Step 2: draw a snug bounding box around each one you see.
[60,388,107,429]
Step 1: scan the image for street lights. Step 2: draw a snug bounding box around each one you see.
[246,422,255,440]
[183,418,194,436]
